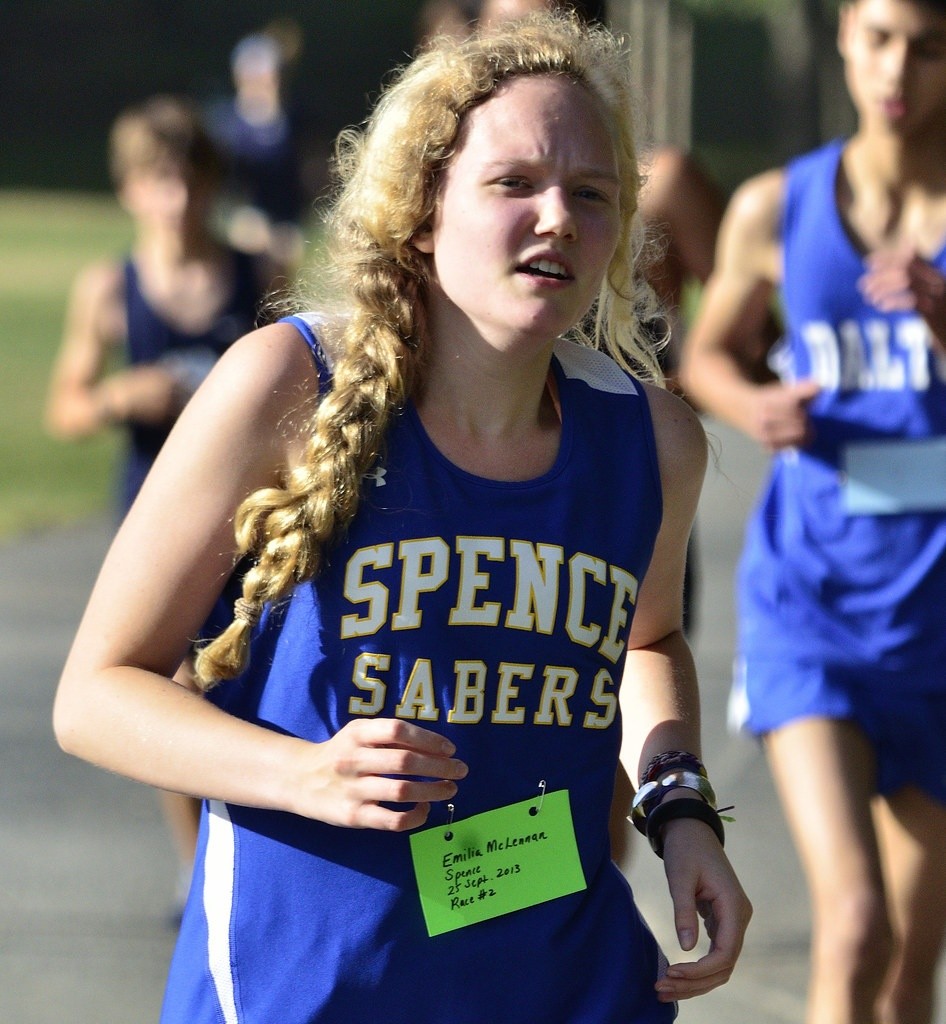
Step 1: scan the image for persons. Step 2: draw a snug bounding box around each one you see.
[681,1,946,1024]
[42,98,315,925]
[420,2,787,642]
[186,24,317,292]
[52,9,753,1023]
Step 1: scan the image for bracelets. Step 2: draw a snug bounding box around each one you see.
[631,751,725,858]
